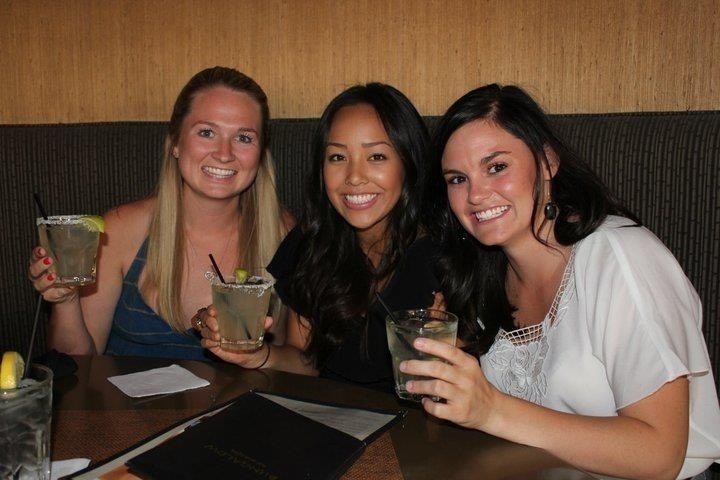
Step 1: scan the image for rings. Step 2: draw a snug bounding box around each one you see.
[197,319,204,329]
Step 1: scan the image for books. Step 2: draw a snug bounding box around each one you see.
[124,393,368,480]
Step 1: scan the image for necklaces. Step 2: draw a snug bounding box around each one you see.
[186,219,235,286]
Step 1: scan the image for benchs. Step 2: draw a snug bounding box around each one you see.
[0,110,720,477]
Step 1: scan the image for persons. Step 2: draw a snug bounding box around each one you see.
[395,83,720,480]
[185,79,511,404]
[26,66,315,381]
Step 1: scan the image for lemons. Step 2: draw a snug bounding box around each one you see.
[78,216,105,234]
[233,269,247,284]
[0,352,25,389]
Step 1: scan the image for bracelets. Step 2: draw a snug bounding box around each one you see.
[253,340,271,369]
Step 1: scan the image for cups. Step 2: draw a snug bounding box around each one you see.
[0,360,55,480]
[208,274,278,353]
[36,213,104,288]
[383,307,460,408]
